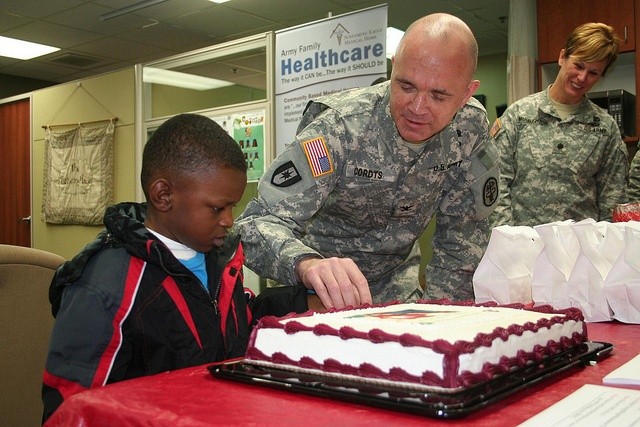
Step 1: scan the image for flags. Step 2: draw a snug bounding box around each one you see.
[301,136,334,176]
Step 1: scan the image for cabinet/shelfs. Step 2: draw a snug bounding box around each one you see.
[536,0,639,147]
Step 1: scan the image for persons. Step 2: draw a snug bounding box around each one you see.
[40,113,329,427]
[487,23,629,228]
[219,12,500,309]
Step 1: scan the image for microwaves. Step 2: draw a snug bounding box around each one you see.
[587,88,635,135]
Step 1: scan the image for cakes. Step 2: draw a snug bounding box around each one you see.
[245,299,590,393]
